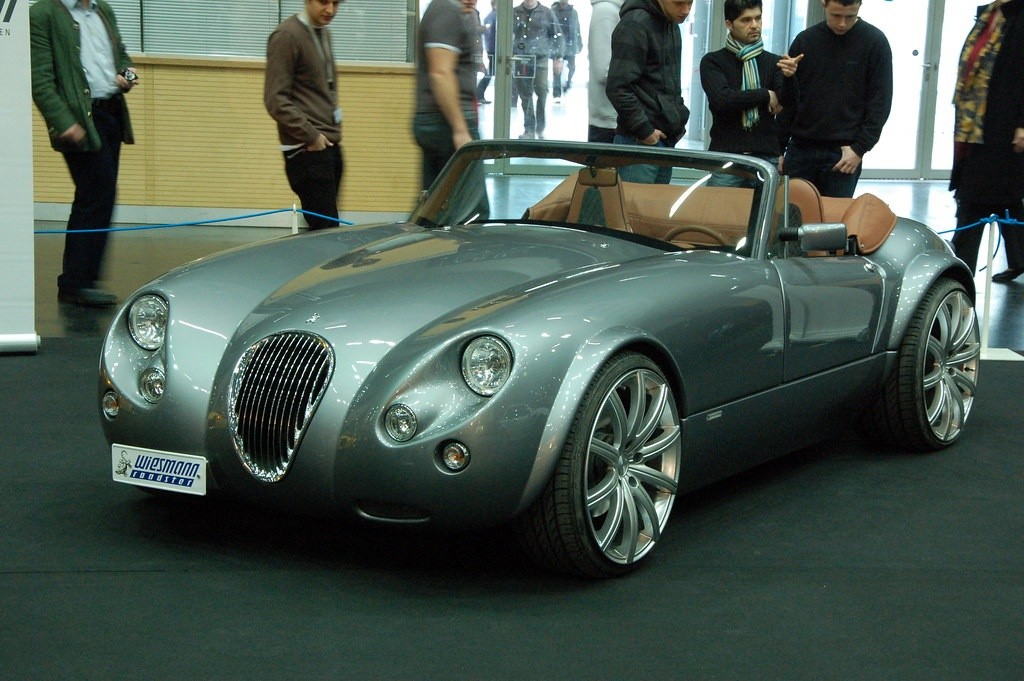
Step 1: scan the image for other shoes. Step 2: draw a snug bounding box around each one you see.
[519,131,535,140]
[553,97,561,103]
[537,132,544,139]
[992,268,1024,284]
[479,98,492,104]
[57,285,117,308]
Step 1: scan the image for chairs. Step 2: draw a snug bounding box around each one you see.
[565,165,634,233]
[766,179,829,256]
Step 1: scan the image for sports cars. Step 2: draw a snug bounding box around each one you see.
[98,138,985,589]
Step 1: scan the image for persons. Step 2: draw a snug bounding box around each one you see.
[586,0,628,149]
[946,0,1024,283]
[700,0,804,190]
[771,0,895,198]
[461,0,584,140]
[264,0,347,229]
[29,0,139,313]
[409,1,494,225]
[606,1,693,187]
